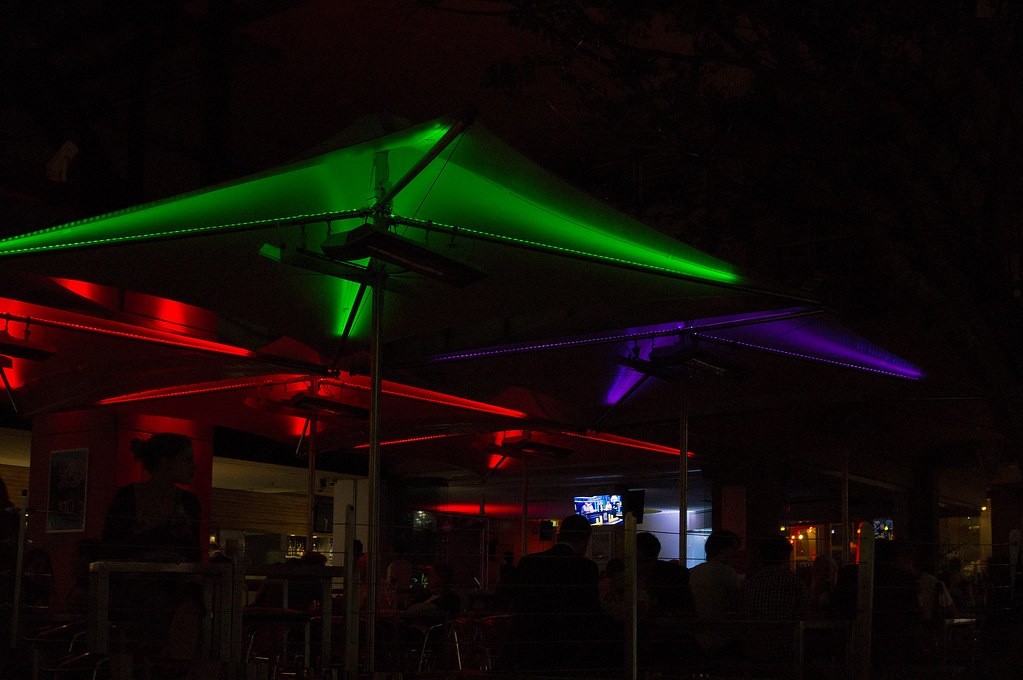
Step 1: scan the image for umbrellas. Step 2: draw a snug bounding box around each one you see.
[0,112,925,650]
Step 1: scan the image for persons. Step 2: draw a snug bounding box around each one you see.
[499,514,693,680]
[261,553,336,655]
[689,531,978,680]
[386,560,447,661]
[68,539,101,602]
[101,434,206,651]
